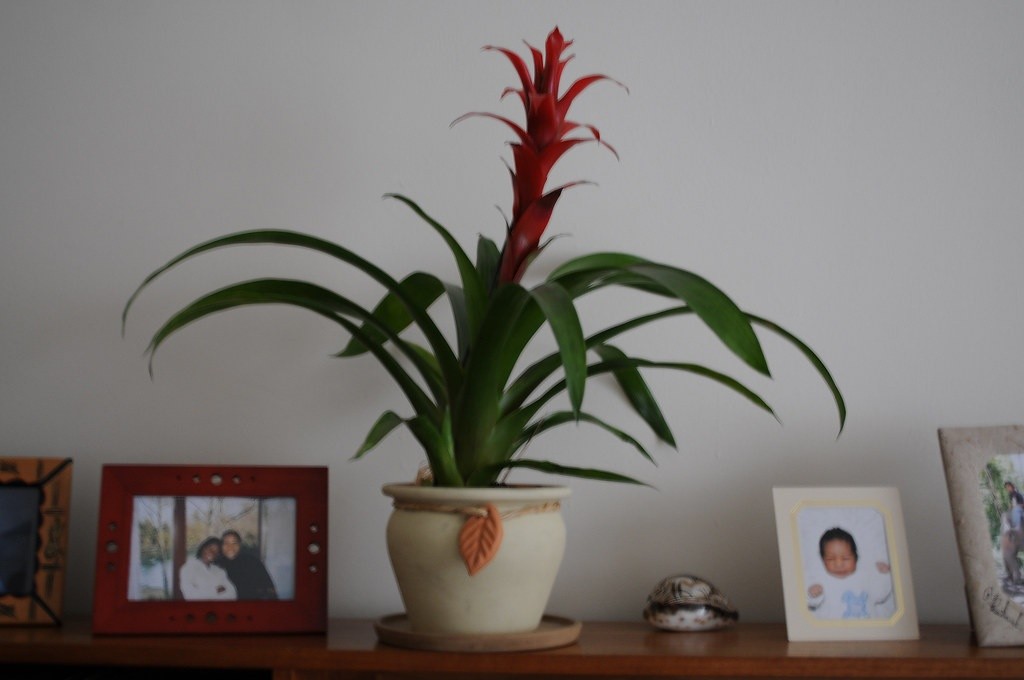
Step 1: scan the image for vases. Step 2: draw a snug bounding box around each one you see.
[382,483,566,634]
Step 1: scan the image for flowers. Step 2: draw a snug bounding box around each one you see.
[120,25,847,489]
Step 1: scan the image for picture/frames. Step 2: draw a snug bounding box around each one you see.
[772,486,917,642]
[0,457,73,623]
[937,426,1024,647]
[93,462,329,635]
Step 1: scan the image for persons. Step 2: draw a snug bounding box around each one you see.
[805,527,894,620]
[179,535,238,599]
[212,529,280,600]
[999,482,1024,583]
